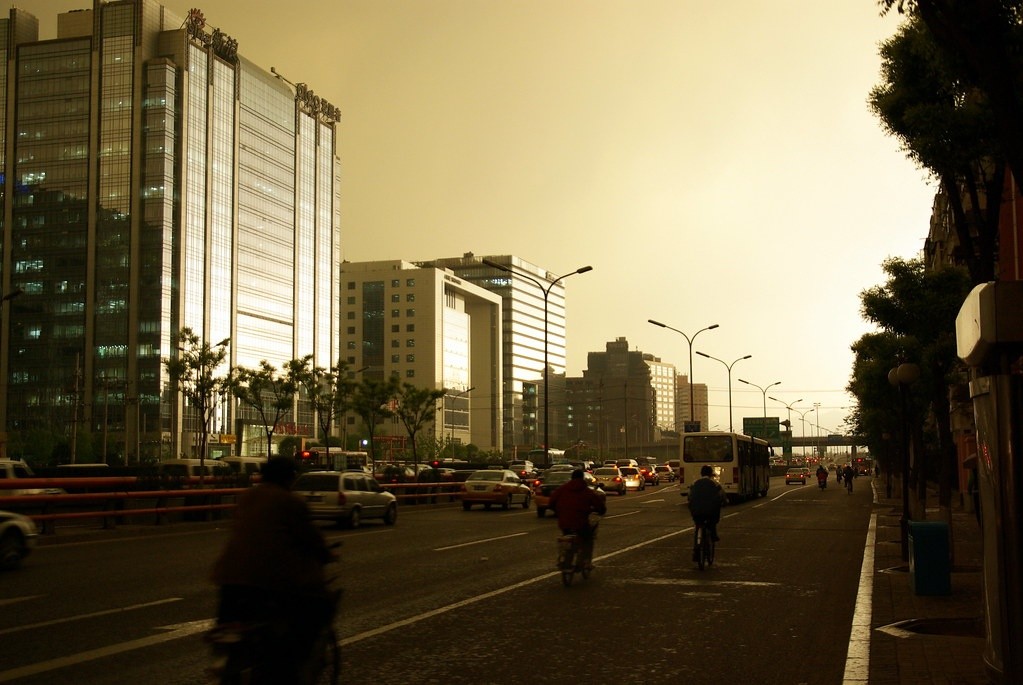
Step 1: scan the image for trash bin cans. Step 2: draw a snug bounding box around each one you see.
[906,520,951,597]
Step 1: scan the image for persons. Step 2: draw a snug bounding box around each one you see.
[548,469,607,571]
[208,454,342,685]
[967,469,982,531]
[686,465,727,563]
[815,465,829,489]
[835,464,879,493]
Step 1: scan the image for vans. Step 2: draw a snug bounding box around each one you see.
[54,455,268,477]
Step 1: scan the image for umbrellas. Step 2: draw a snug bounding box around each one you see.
[962,453,979,469]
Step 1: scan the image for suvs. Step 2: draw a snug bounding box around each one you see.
[291,468,398,529]
[0,456,68,497]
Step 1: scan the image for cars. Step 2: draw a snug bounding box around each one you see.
[771,454,833,486]
[379,463,455,481]
[0,510,39,572]
[458,456,679,512]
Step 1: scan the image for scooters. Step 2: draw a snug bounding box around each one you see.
[837,474,853,492]
[557,483,605,588]
[818,480,827,491]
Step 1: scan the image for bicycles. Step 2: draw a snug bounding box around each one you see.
[692,518,716,571]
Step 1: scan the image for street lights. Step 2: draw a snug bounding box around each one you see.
[695,351,752,433]
[888,364,917,562]
[647,318,719,422]
[737,378,834,465]
[175,336,474,474]
[482,256,593,469]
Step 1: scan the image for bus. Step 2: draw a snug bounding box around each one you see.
[679,431,775,504]
[301,447,369,473]
[528,449,570,467]
[856,457,873,476]
